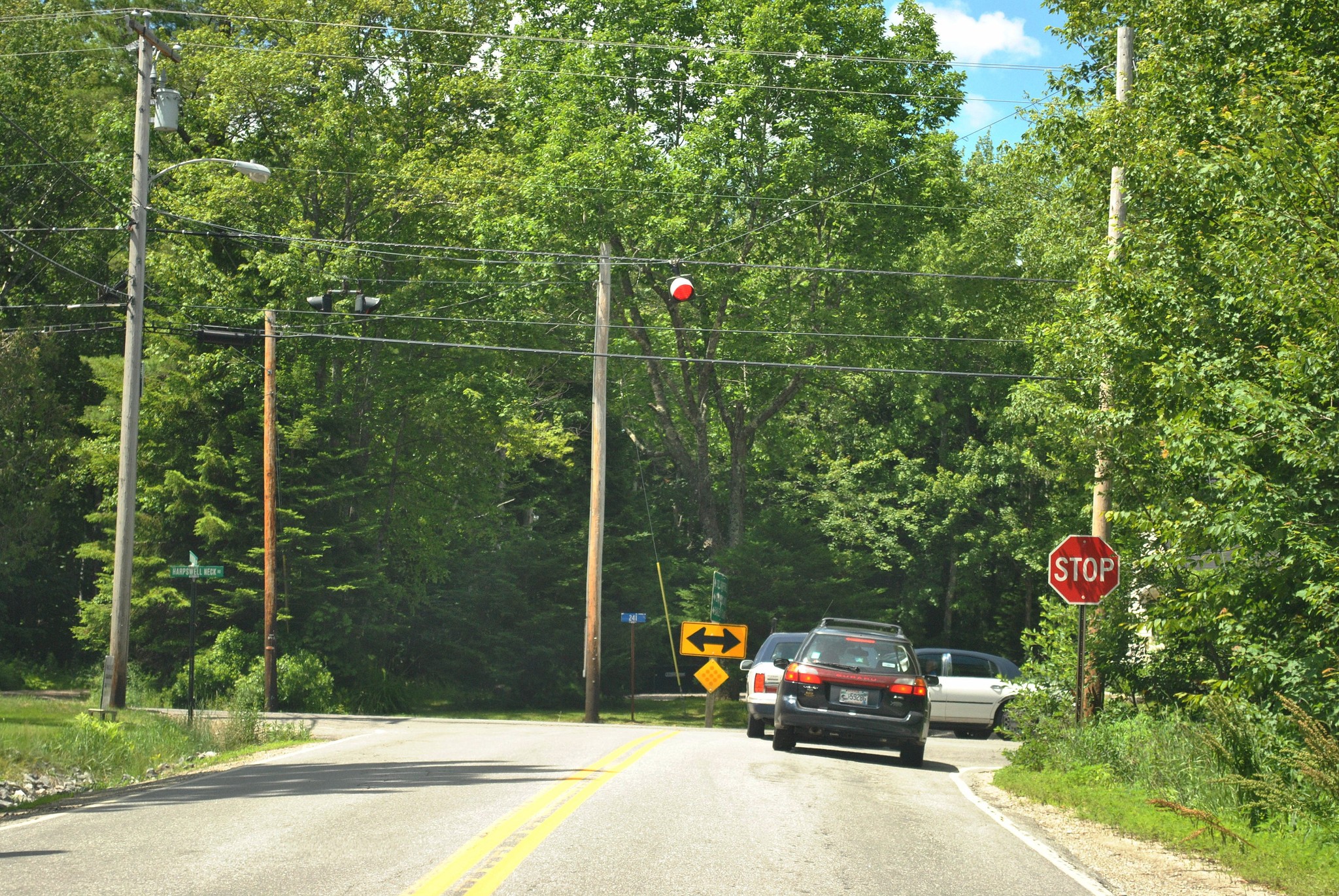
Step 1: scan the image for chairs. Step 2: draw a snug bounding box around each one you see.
[874,658,899,674]
[818,649,844,663]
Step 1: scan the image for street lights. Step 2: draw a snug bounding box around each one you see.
[103,158,270,708]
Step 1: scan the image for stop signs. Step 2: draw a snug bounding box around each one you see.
[1049,537,1121,606]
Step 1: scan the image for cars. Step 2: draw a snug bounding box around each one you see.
[740,631,847,737]
[884,649,1079,741]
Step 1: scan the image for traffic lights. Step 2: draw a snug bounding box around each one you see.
[307,294,332,314]
[355,294,384,313]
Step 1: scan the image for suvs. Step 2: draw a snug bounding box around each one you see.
[771,620,940,767]
